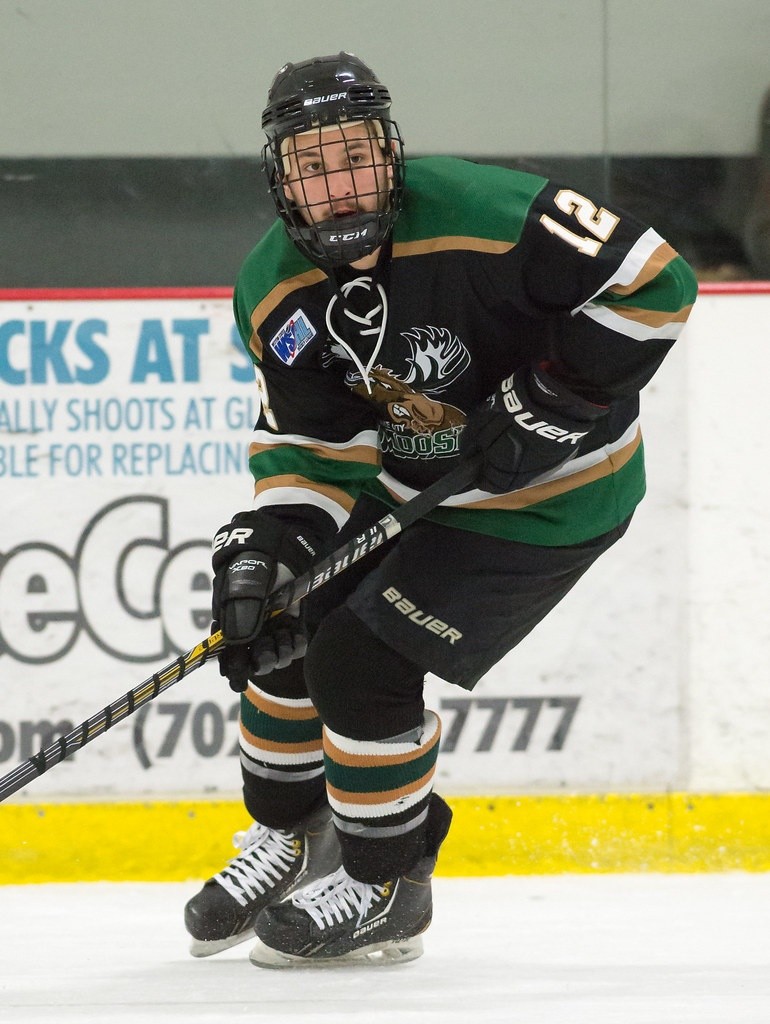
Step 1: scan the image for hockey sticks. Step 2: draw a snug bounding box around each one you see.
[0,463,480,800]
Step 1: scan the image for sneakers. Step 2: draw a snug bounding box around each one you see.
[250,794,452,969]
[184,786,342,959]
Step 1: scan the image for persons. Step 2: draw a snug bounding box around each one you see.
[183,55,699,971]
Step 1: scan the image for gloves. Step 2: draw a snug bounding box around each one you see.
[211,510,315,693]
[458,359,610,495]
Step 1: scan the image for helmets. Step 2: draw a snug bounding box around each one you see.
[261,50,407,264]
